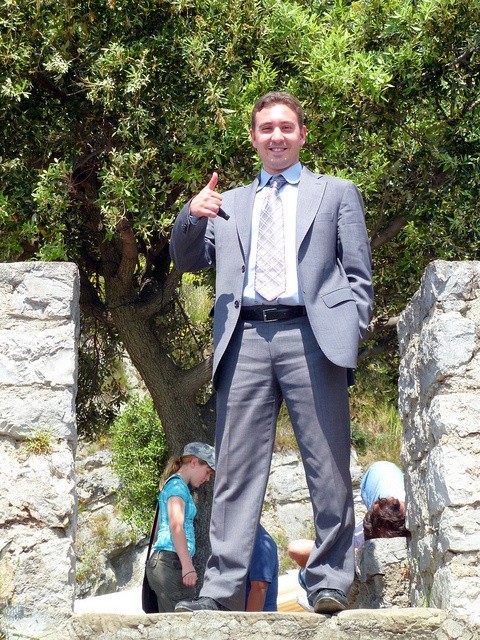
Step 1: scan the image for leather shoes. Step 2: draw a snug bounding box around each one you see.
[313,588,348,612]
[173,596,227,611]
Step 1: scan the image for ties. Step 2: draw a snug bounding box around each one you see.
[253,174,286,302]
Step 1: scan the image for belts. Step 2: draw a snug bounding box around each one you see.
[241,304,306,322]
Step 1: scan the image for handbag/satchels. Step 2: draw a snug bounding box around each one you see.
[142,573,159,614]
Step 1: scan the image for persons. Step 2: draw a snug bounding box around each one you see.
[246,522,280,613]
[169,91,376,612]
[287,496,410,590]
[145,442,216,612]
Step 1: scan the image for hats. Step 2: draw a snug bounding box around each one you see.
[182,442,215,471]
